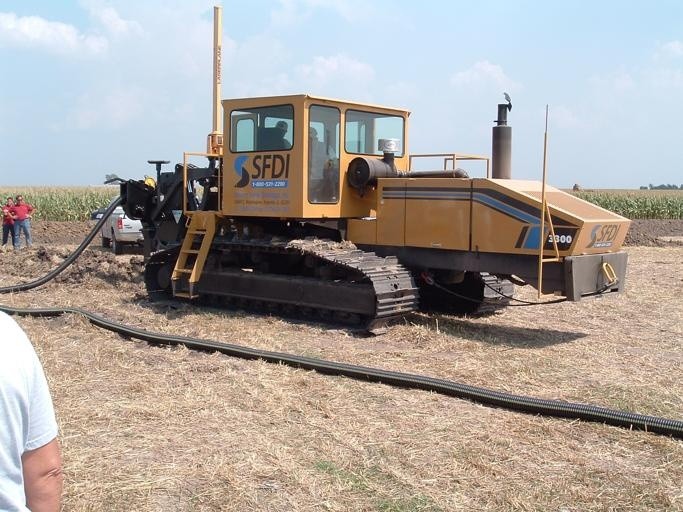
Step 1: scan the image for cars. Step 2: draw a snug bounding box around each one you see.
[94,198,145,251]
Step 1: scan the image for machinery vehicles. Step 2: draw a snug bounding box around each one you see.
[118,7,633,325]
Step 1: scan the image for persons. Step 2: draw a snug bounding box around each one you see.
[2,197,14,246]
[276,120,291,151]
[0,310,63,512]
[8,195,35,251]
